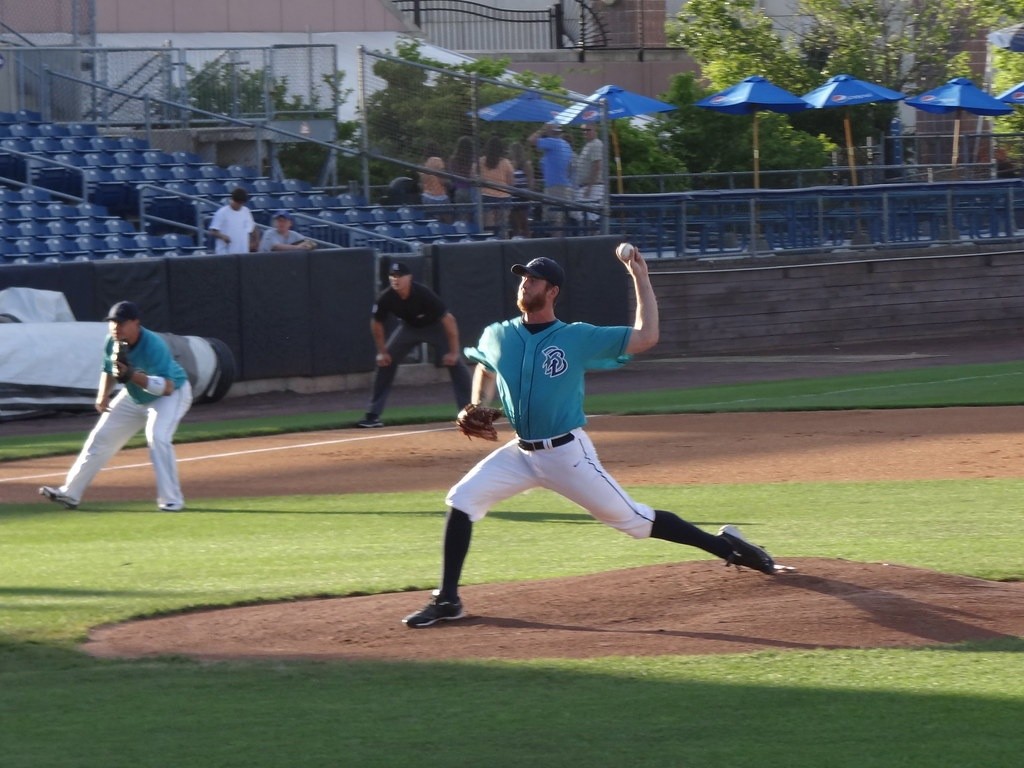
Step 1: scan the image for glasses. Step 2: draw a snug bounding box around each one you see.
[582,128,592,131]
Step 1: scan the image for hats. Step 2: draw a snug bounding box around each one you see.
[510,257,565,289]
[388,263,410,276]
[273,210,290,219]
[105,301,140,323]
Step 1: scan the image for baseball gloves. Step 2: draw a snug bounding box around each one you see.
[111,337,134,384]
[455,403,507,444]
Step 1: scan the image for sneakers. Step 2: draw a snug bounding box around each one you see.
[719,525,775,575]
[401,592,462,628]
[39,485,78,509]
[359,412,383,427]
[160,501,183,513]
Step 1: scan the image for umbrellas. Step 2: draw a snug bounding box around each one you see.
[987,23,1024,52]
[692,76,817,238]
[553,84,679,192]
[996,83,1024,104]
[904,77,1016,175]
[802,75,907,231]
[463,92,566,123]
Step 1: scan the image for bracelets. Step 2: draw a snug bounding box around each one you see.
[118,367,134,383]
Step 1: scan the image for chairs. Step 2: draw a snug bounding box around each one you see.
[0,112,525,267]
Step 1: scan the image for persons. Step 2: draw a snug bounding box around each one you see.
[480,137,515,238]
[40,302,192,511]
[258,211,312,252]
[356,262,472,427]
[509,142,532,235]
[527,123,603,236]
[993,149,1015,178]
[402,247,775,626]
[421,136,476,221]
[210,188,259,253]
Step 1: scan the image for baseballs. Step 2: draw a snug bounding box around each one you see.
[617,242,634,261]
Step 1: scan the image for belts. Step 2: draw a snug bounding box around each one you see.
[578,182,604,189]
[517,432,574,451]
